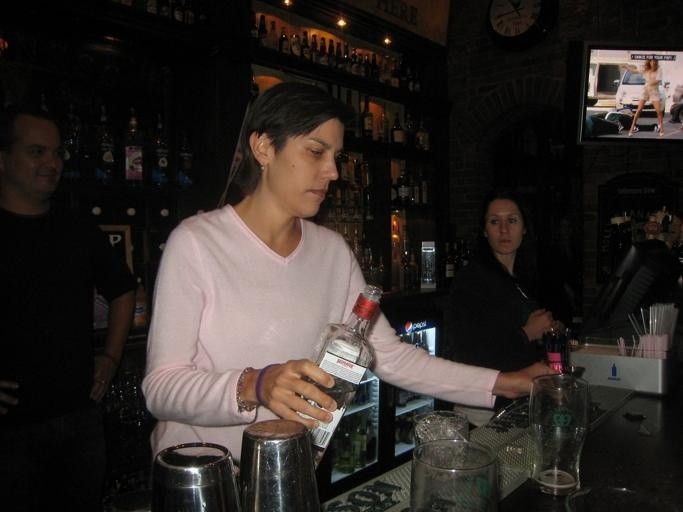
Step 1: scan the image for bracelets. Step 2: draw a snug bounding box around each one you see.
[236,367,256,411]
[255,364,280,409]
[99,350,116,364]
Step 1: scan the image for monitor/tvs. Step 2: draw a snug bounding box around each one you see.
[576,41,683,146]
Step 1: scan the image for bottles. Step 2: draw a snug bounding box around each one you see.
[315,151,470,291]
[328,86,430,152]
[120,0,207,25]
[133,278,146,327]
[291,284,383,473]
[105,370,149,430]
[2,88,170,191]
[249,11,422,94]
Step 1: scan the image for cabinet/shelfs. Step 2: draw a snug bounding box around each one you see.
[1,1,234,386]
[233,1,452,359]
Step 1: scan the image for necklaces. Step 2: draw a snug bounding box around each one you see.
[235,206,301,258]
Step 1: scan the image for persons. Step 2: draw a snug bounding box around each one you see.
[449,191,574,411]
[621,59,666,138]
[138,81,558,512]
[0,104,135,511]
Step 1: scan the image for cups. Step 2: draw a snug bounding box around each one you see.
[151,442,239,512]
[412,410,469,459]
[410,440,499,512]
[529,376,589,495]
[546,329,576,375]
[240,419,321,512]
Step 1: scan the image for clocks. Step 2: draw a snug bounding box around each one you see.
[485,0,558,54]
